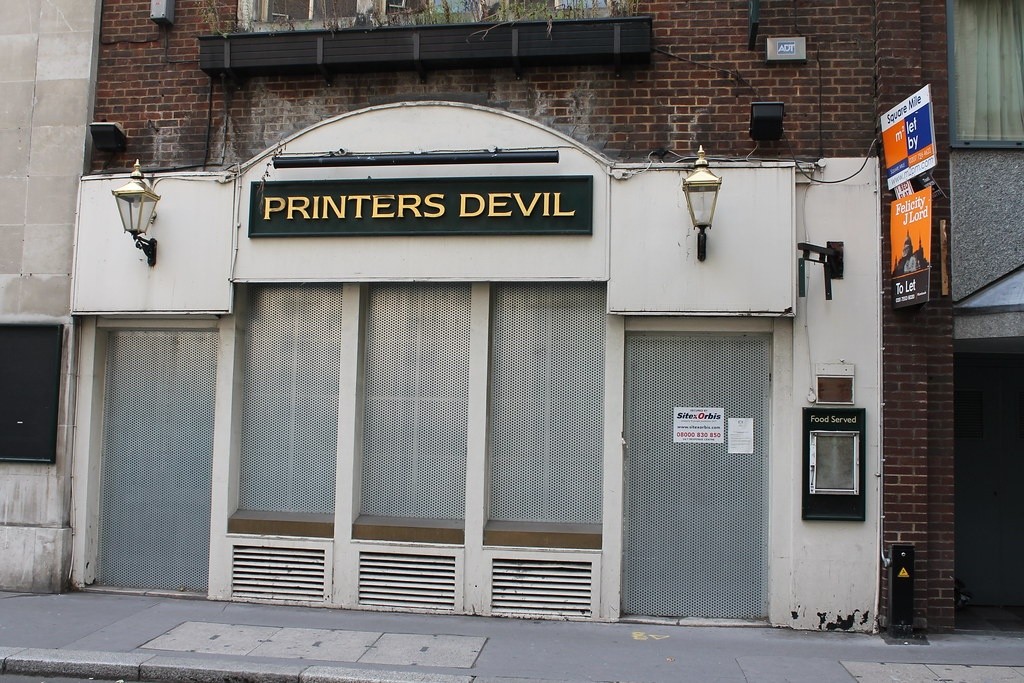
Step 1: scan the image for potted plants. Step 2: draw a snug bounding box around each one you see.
[195,0,653,70]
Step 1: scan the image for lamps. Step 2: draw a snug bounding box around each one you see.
[111,158,162,266]
[749,101,786,141]
[682,144,722,262]
[89,121,127,153]
